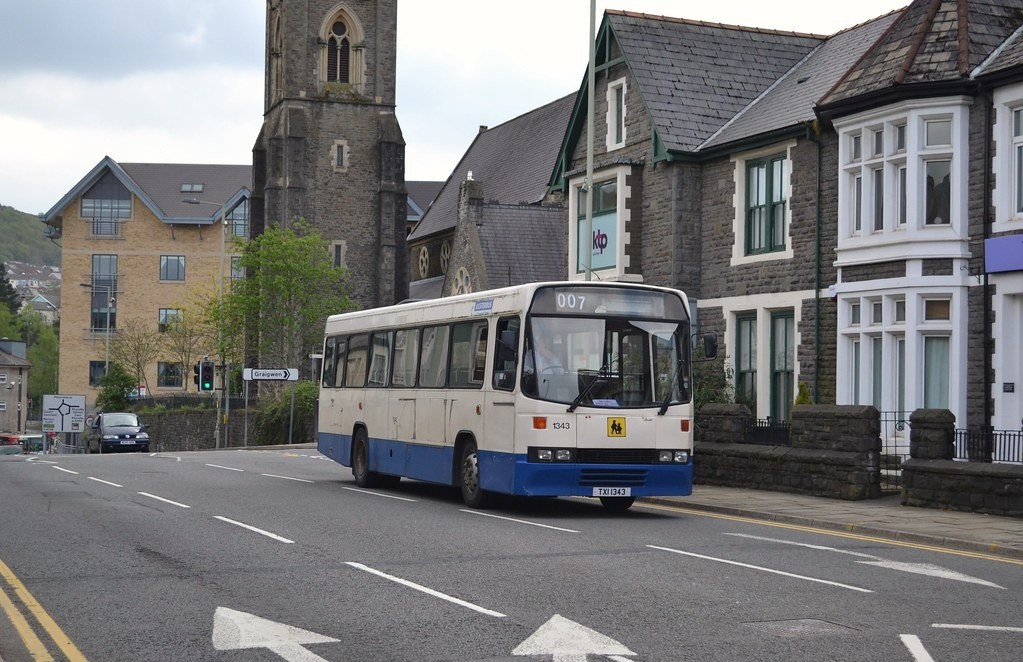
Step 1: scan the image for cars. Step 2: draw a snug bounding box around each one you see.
[87,411,151,453]
[47,432,58,443]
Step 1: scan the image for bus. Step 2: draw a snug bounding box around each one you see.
[315,281,719,510]
[20,436,49,455]
[0,435,19,445]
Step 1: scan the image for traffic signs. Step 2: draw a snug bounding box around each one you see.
[243,369,299,380]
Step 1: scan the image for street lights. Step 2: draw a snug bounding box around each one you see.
[181,198,226,449]
[79,283,110,386]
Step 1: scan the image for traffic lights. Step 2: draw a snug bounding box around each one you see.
[28,399,32,409]
[195,361,214,392]
